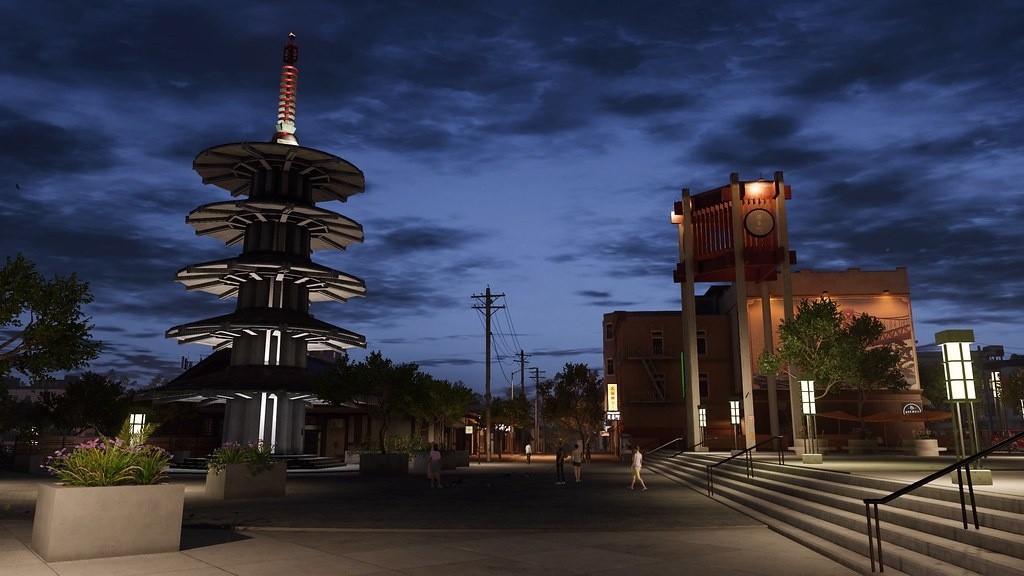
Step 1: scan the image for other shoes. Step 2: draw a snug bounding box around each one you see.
[627,486,633,489]
[431,486,434,488]
[555,481,561,484]
[576,480,581,483]
[437,485,442,487]
[561,482,565,484]
[642,487,647,490]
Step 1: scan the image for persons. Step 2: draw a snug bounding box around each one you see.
[630,445,648,490]
[569,443,582,482]
[554,441,566,484]
[584,443,592,464]
[428,443,444,488]
[525,441,532,464]
